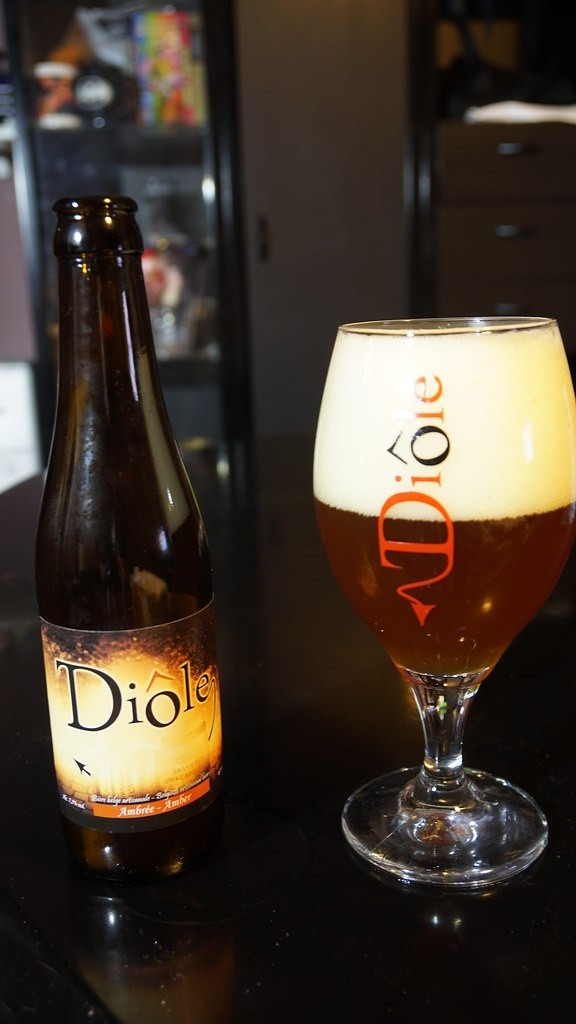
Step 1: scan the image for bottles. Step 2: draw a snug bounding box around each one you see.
[57,803,240,1024]
[34,196,232,833]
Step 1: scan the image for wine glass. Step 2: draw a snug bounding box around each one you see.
[313,315,576,888]
